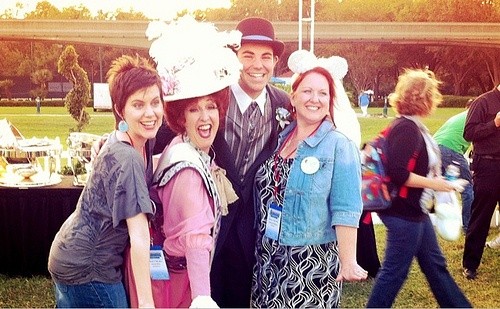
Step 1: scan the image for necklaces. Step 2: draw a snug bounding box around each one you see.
[126,132,146,160]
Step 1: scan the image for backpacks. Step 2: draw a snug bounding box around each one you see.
[358,118,421,211]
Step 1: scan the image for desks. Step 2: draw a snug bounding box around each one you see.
[0,173,86,279]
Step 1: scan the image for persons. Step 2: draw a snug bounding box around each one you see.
[462,83,500,281]
[364,64,472,309]
[253,49,369,309]
[146,18,298,309]
[122,14,242,309]
[431,97,474,236]
[358,90,370,118]
[48,52,163,309]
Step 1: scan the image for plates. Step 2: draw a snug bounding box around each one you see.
[0,163,63,188]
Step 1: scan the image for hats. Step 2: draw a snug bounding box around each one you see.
[145,14,242,103]
[236,18,285,58]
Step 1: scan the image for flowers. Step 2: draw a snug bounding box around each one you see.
[276,107,291,130]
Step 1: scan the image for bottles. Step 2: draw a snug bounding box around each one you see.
[438,161,461,205]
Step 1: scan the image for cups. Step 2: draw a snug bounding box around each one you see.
[34,156,53,185]
[70,150,91,187]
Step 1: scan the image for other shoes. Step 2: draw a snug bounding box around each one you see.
[463,268,476,279]
[486,238,500,250]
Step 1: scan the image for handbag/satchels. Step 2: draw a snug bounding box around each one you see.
[435,192,462,241]
